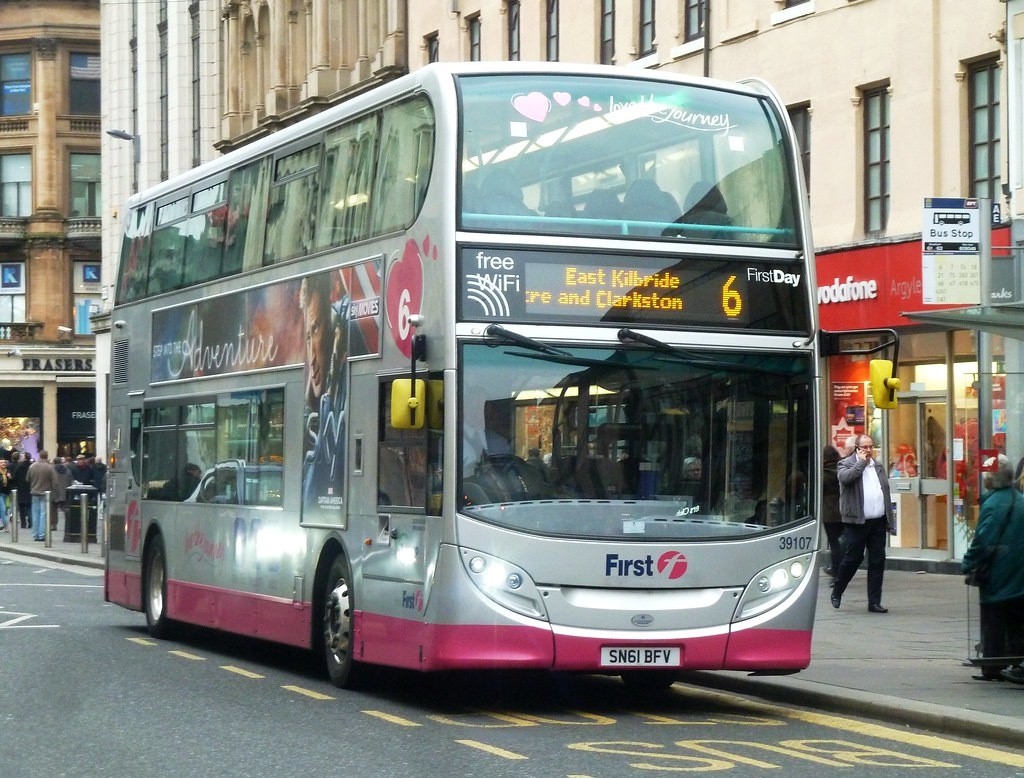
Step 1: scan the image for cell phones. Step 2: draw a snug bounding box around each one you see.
[854,446,860,453]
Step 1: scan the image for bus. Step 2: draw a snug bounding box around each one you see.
[104,61,901,689]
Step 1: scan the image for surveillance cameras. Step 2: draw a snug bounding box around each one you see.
[58,326,73,332]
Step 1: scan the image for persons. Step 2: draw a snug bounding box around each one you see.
[0,449,107,541]
[822,446,842,576]
[960,454,1024,683]
[163,463,203,490]
[683,457,702,480]
[300,272,347,451]
[832,434,896,613]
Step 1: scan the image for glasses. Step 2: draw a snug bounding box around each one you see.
[857,445,874,450]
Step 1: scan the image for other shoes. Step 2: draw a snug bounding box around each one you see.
[831,595,841,608]
[1000,665,1024,684]
[972,670,997,680]
[868,603,888,613]
[823,566,835,577]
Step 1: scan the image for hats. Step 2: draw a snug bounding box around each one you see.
[54,457,61,464]
[40,450,48,459]
[76,455,85,460]
[12,452,19,460]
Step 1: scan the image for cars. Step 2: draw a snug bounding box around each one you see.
[183,463,308,569]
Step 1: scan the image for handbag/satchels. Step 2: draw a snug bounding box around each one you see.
[6,491,13,508]
[965,549,998,587]
